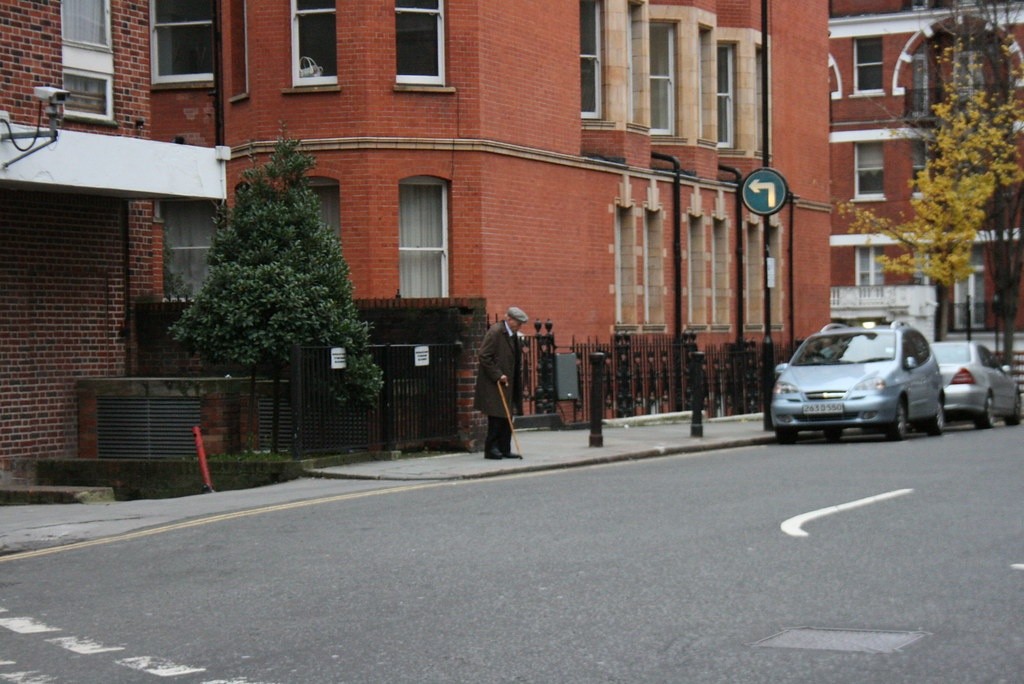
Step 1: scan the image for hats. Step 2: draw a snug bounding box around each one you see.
[508,307,528,323]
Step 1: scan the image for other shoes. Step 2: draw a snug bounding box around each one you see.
[485,448,503,459]
[503,450,519,459]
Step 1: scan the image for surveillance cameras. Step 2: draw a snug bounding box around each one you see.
[33,86,71,105]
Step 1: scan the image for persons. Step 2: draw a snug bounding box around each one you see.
[472,306,528,459]
[807,338,844,360]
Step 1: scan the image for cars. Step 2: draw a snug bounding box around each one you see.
[769,322,947,441]
[927,340,1023,429]
